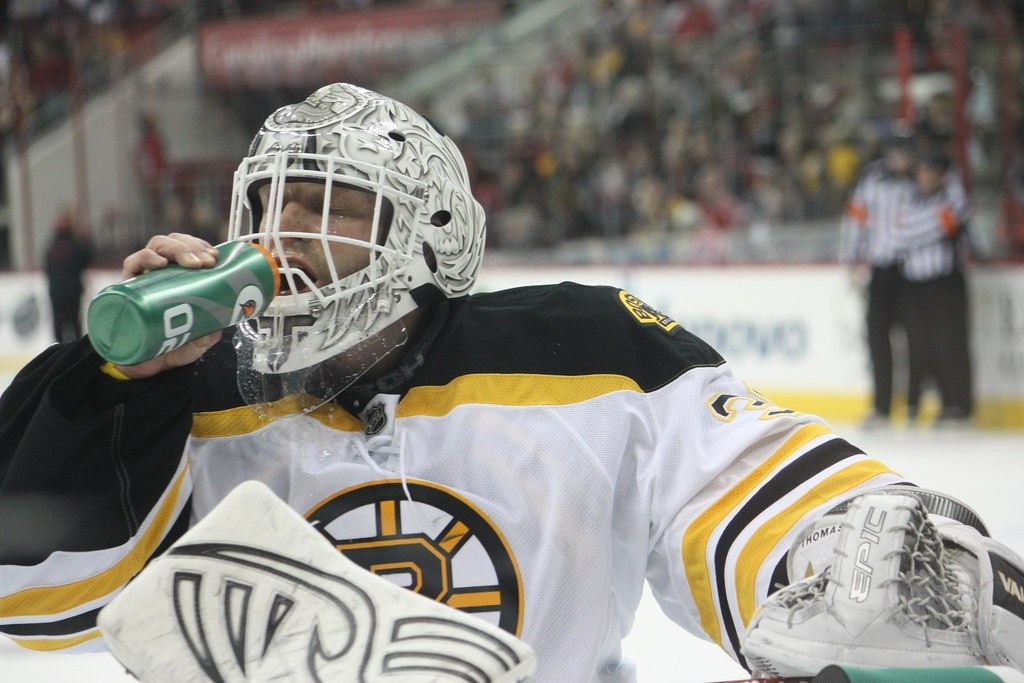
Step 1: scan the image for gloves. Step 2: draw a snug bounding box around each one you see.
[741,493,1024,677]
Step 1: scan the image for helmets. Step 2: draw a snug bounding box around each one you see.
[229,81,488,375]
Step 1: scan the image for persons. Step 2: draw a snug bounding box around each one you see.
[94,1,1024,264]
[902,150,972,424]
[44,209,92,343]
[1,84,1024,682]
[839,135,960,423]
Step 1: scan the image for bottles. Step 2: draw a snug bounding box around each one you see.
[87,238,282,367]
